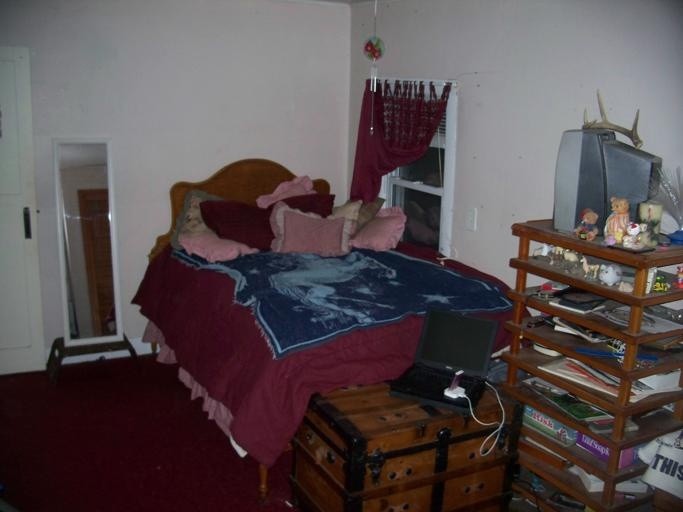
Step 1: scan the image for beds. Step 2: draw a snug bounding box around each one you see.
[130,159,531,502]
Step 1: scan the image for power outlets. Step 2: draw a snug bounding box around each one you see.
[464,207,477,232]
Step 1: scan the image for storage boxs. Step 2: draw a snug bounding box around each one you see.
[288,372,509,512]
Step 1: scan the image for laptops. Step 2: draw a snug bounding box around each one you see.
[389,307,499,415]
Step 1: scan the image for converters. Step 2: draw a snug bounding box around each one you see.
[444,386,465,398]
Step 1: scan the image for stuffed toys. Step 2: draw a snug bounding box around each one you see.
[604,197,629,244]
[575,212,598,241]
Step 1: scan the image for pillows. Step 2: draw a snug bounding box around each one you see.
[270,202,351,257]
[358,198,384,230]
[200,194,335,250]
[179,226,258,262]
[328,200,362,219]
[351,207,406,250]
[258,176,317,209]
[172,190,226,249]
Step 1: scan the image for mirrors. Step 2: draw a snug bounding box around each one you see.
[53,136,123,346]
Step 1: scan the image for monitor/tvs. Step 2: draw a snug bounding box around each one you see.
[553,127,663,235]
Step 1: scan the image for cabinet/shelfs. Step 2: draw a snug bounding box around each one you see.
[371,77,457,259]
[499,219,683,512]
